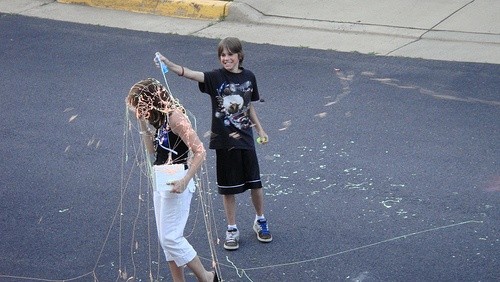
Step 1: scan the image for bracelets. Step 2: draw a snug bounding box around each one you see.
[140,128,150,136]
[178,66,184,76]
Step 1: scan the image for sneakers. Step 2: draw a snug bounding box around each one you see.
[253,217,273,242]
[224,226,240,250]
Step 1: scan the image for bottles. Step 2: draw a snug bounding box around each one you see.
[155,55,169,74]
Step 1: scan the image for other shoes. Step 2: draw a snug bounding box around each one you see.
[211,271,221,282]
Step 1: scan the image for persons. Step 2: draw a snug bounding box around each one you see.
[153,37,273,250]
[125,78,219,282]
